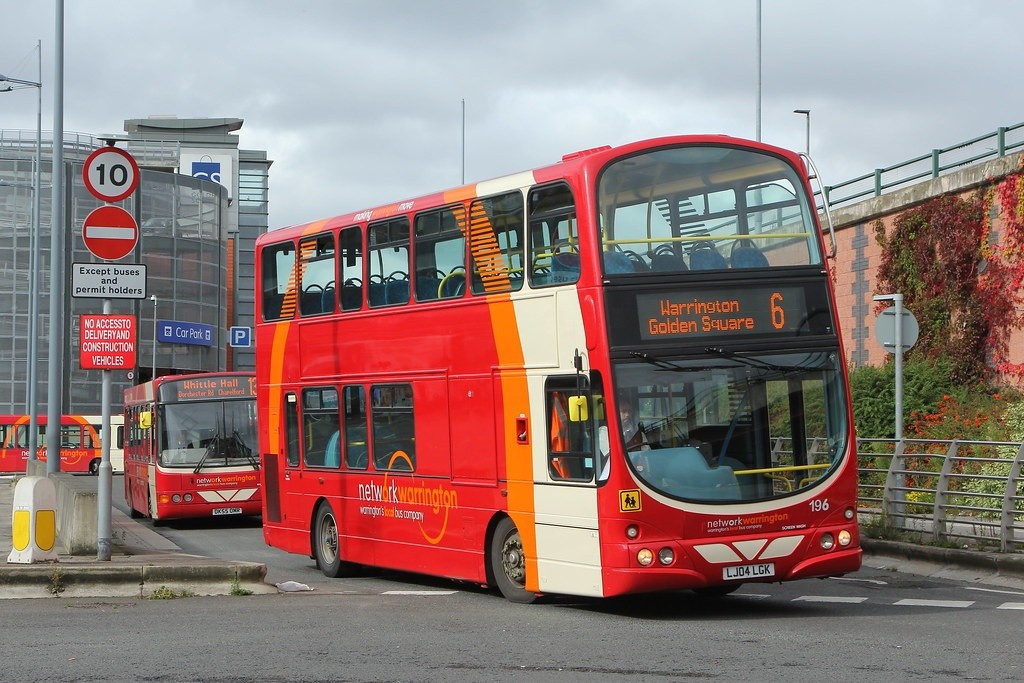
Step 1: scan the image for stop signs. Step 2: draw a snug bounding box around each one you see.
[82,205,139,261]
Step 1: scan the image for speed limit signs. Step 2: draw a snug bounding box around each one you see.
[83,146,140,202]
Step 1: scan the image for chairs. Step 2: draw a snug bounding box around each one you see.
[268,237,770,322]
[300,415,415,474]
[573,420,594,478]
[0,442,80,449]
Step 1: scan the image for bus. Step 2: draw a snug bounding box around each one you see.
[124,371,263,527]
[0,414,124,476]
[255,134,864,604]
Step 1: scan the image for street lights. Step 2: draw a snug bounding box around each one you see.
[793,108,811,176]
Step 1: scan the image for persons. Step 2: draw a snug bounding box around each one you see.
[174,431,193,448]
[599,400,650,462]
